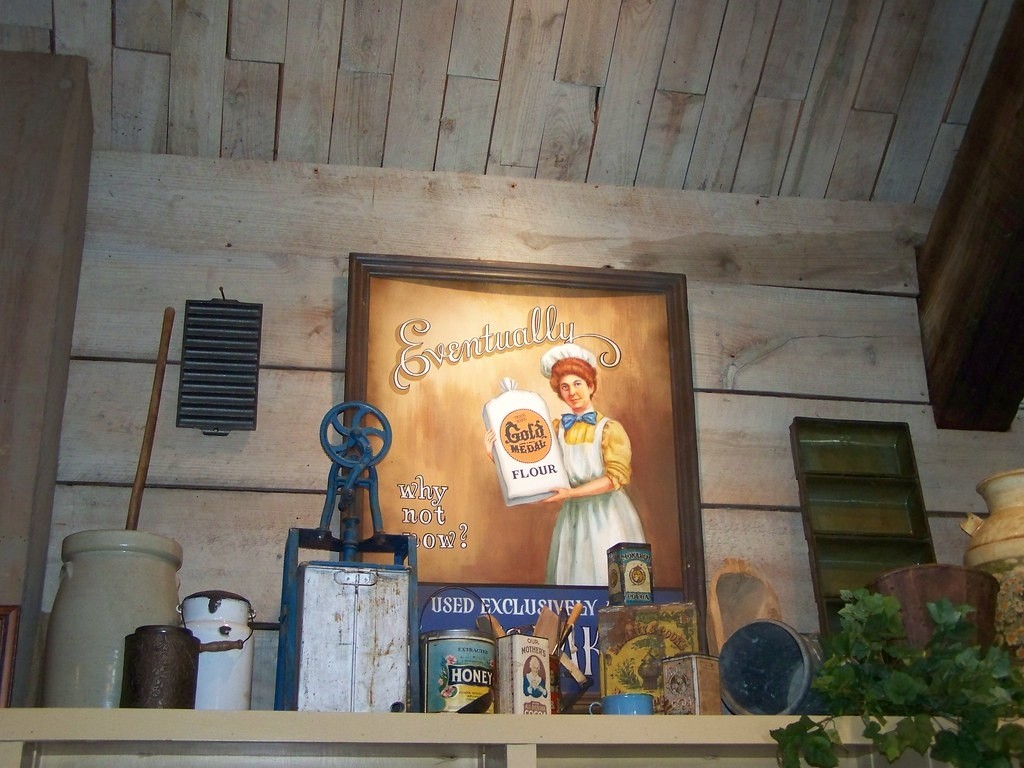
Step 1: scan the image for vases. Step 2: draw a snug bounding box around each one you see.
[960,469,1024,675]
[864,561,1001,671]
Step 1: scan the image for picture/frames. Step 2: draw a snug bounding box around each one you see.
[341,252,707,714]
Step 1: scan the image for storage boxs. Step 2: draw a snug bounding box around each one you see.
[597,602,700,715]
[607,542,655,607]
[662,652,723,716]
[495,634,552,715]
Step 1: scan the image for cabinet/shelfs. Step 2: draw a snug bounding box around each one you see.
[0,705,1024,768]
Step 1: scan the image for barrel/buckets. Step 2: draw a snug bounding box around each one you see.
[176,590,256,710]
[718,619,828,716]
[418,585,496,714]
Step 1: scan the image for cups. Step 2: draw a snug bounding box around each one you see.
[589,693,654,715]
[550,654,560,714]
